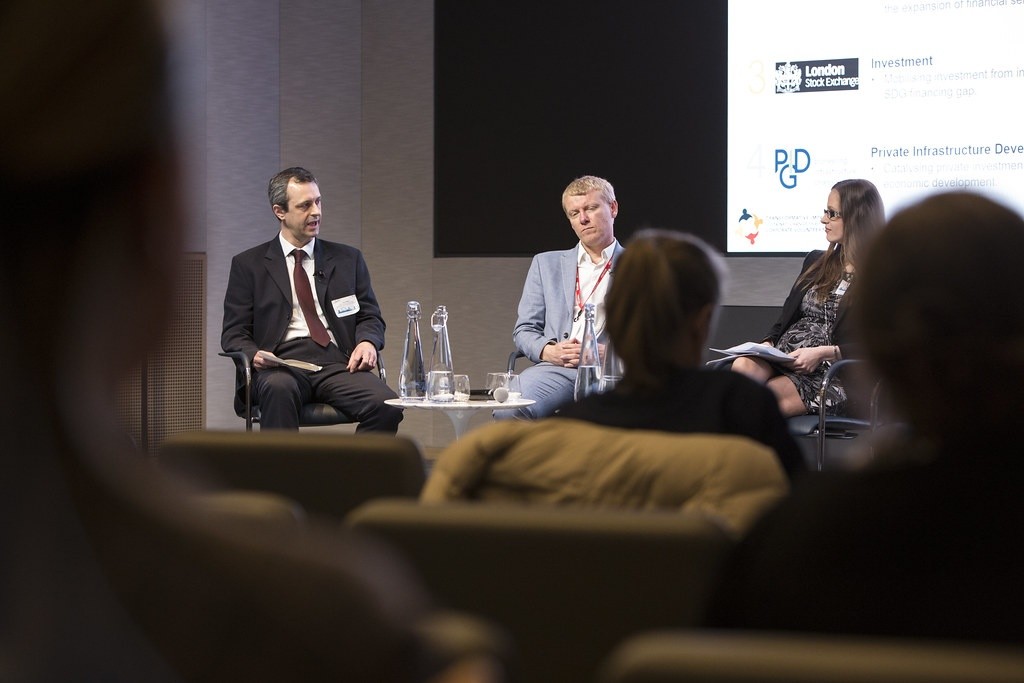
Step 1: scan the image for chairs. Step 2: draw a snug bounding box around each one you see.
[145,479,343,586]
[346,497,735,683]
[506,346,536,387]
[594,626,1023,683]
[151,425,428,543]
[706,353,882,473]
[252,611,507,683]
[217,347,387,435]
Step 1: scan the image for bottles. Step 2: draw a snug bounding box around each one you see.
[427,304,455,402]
[600,331,624,396]
[571,302,602,407]
[397,300,429,405]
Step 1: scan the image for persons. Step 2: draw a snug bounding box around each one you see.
[730,178,887,420]
[0,3,503,683]
[222,168,404,436]
[562,230,806,478]
[707,191,1023,632]
[494,176,626,423]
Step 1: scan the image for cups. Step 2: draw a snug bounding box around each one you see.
[454,374,471,403]
[486,372,508,401]
[494,373,521,402]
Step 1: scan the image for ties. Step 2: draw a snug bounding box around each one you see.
[289,248,331,347]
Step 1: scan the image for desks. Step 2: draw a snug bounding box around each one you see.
[385,393,537,444]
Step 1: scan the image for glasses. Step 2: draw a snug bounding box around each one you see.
[823,209,841,220]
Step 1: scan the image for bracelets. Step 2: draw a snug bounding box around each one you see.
[833,346,838,361]
